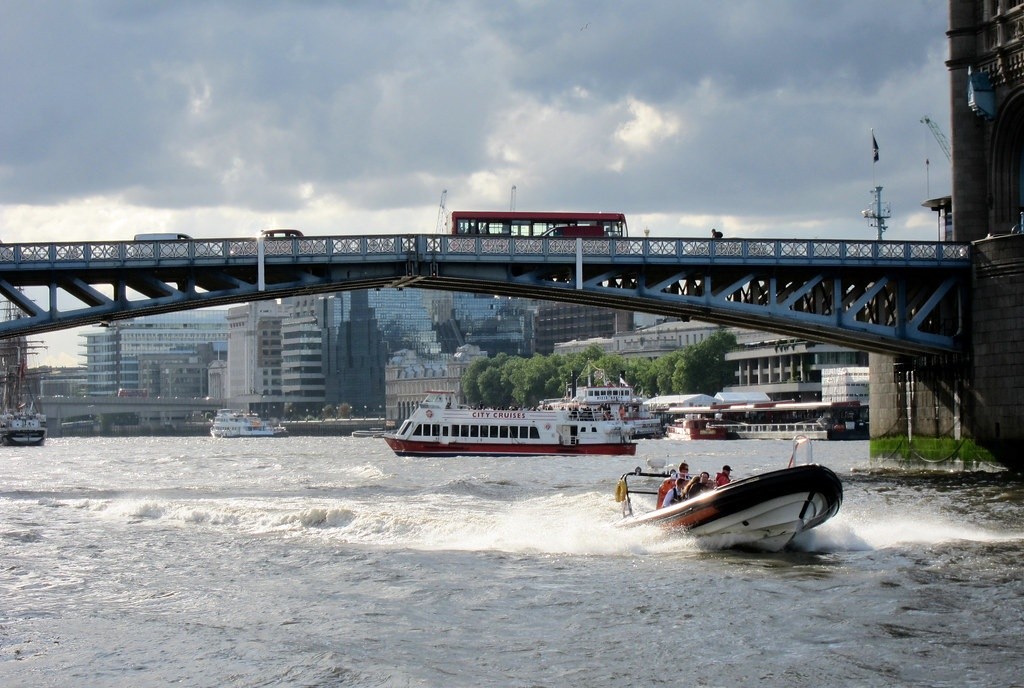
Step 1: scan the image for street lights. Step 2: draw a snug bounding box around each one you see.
[289,409,292,423]
[363,405,367,421]
[349,405,353,420]
[378,404,382,420]
[305,408,309,422]
[336,406,339,421]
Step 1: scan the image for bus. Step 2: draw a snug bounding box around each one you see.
[450,211,630,238]
[118,388,150,398]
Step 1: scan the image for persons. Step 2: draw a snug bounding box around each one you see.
[712,229,722,239]
[660,462,733,508]
[472,400,613,421]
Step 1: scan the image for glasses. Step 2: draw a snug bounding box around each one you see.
[684,469,688,470]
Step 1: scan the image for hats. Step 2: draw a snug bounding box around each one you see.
[723,465,732,471]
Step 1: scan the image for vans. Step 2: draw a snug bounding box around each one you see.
[259,229,304,238]
[133,233,192,241]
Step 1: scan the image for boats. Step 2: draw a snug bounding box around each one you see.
[664,397,869,441]
[537,357,666,441]
[209,407,276,437]
[381,388,642,457]
[612,435,845,556]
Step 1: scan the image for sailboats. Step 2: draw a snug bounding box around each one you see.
[0,284,49,447]
